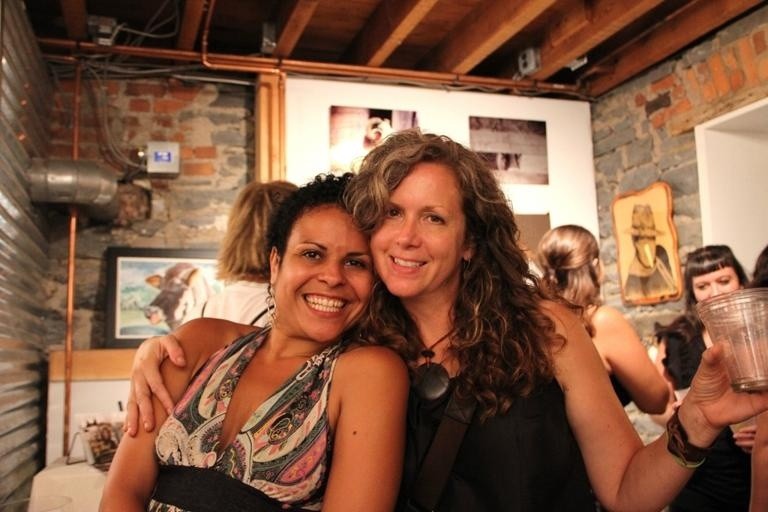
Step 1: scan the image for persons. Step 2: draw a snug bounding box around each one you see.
[124,128,768,512]
[98,173,410,512]
[535,224,670,416]
[180,181,299,329]
[657,245,768,512]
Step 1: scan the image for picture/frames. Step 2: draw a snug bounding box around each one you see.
[612,181,683,307]
[105,246,221,350]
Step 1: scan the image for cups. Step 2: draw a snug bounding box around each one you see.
[695,287,768,392]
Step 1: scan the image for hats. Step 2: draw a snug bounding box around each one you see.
[624,204,667,236]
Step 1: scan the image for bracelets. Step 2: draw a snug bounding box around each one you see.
[665,405,716,468]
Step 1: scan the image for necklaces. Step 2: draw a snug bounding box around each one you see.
[415,327,457,400]
